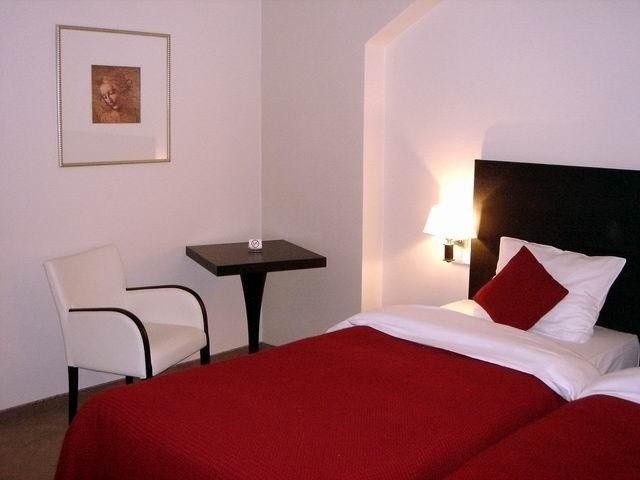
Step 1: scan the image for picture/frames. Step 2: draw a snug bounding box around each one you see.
[56,24,171,167]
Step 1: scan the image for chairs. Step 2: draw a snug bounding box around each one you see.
[41,245,212,427]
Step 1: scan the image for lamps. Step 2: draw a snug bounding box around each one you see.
[423,204,469,263]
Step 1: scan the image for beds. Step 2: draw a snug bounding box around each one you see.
[56,159,640,480]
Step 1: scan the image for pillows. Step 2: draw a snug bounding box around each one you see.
[471,245,568,332]
[496,236,626,343]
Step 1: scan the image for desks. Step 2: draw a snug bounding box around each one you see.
[186,238,326,353]
